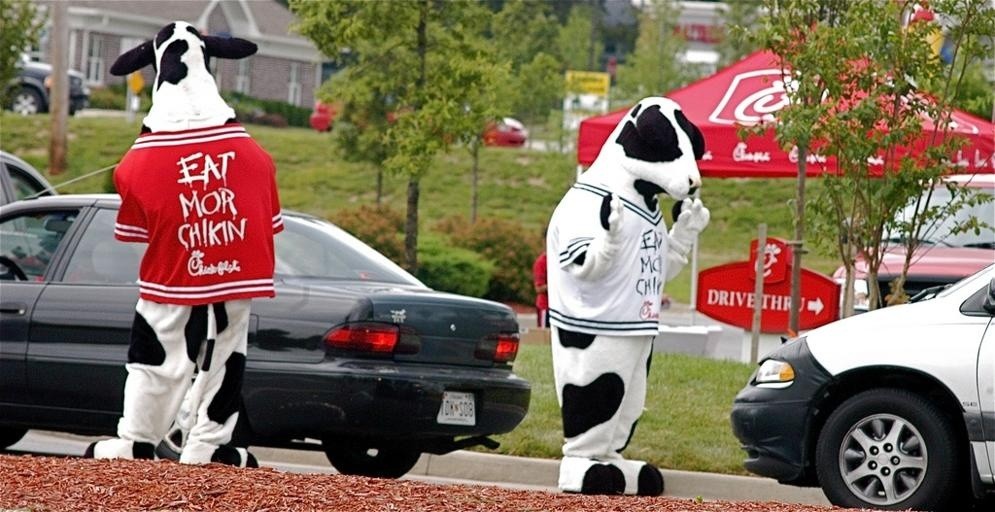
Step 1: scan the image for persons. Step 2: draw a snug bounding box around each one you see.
[531,228,553,329]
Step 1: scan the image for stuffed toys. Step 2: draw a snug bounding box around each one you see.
[543,95,712,498]
[77,19,288,472]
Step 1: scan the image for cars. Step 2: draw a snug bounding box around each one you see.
[311,79,527,146]
[0,48,88,118]
